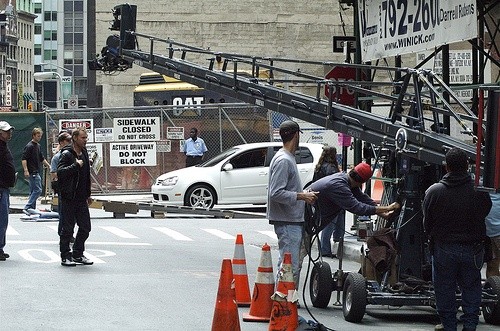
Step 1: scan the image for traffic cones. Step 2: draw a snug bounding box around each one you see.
[241,242,275,321]
[210,259,242,331]
[267,252,299,331]
[231,234,252,305]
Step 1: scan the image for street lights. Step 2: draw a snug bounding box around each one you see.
[38,59,75,112]
[34,71,65,110]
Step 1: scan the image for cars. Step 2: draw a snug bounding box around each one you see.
[151,142,325,212]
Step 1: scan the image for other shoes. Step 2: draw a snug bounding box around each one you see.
[73,255,94,265]
[0,249,10,261]
[322,253,336,257]
[333,237,344,242]
[434,323,457,331]
[60,258,77,266]
[461,324,476,331]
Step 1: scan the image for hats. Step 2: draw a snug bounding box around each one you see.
[355,162,372,180]
[58,132,72,142]
[0,120,16,132]
[279,120,304,134]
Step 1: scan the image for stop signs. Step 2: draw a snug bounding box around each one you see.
[322,65,369,107]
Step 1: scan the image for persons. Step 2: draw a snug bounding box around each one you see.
[22,127,51,216]
[266,120,401,308]
[423,147,493,331]
[183,128,208,168]
[50,127,93,266]
[0,121,15,261]
